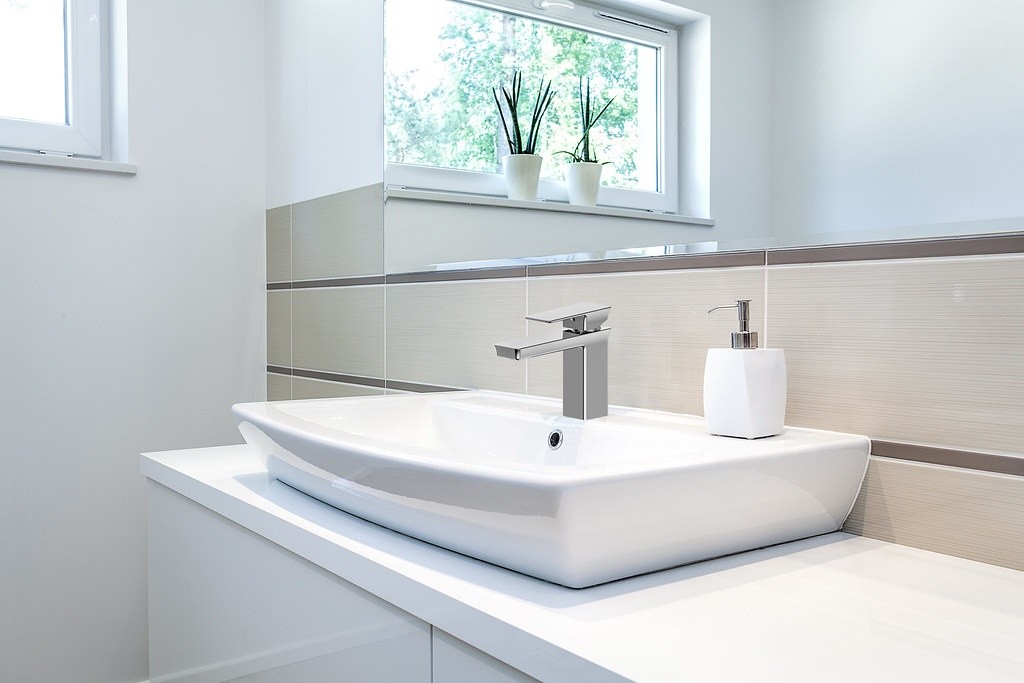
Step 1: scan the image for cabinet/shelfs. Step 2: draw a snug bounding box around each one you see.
[140,444,1024,683]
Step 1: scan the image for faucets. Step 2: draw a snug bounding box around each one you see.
[494,302,610,420]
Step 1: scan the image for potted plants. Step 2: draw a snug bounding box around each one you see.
[551,74,617,207]
[493,70,558,200]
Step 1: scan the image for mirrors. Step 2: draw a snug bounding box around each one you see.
[381,0,1024,276]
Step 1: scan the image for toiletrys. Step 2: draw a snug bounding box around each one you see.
[705,295,788,437]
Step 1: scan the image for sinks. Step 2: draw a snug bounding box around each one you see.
[232,386,872,590]
